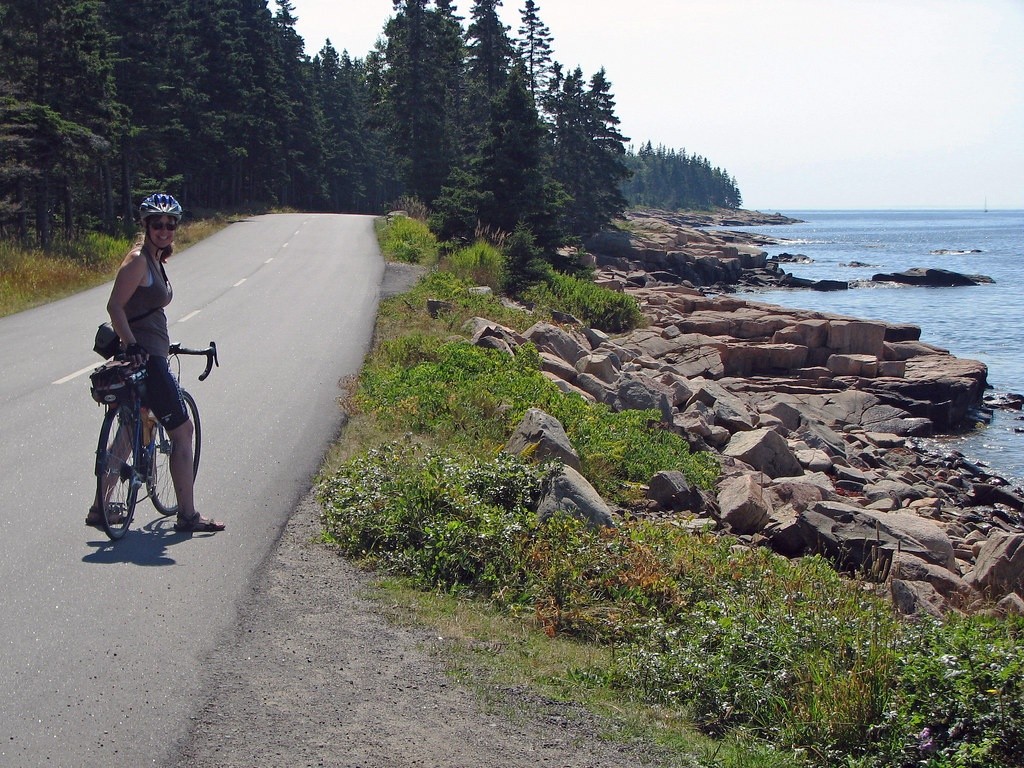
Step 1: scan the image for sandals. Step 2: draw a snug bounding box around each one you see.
[173,511,226,532]
[85,506,135,525]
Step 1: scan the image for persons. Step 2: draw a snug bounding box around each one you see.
[85,193,224,532]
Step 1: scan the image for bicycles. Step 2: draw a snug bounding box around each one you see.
[95,341,220,540]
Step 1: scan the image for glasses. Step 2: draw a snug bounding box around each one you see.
[149,221,177,231]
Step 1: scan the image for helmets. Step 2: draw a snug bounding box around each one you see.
[139,194,183,222]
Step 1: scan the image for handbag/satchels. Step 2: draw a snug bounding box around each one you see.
[93,321,120,360]
[89,352,149,404]
